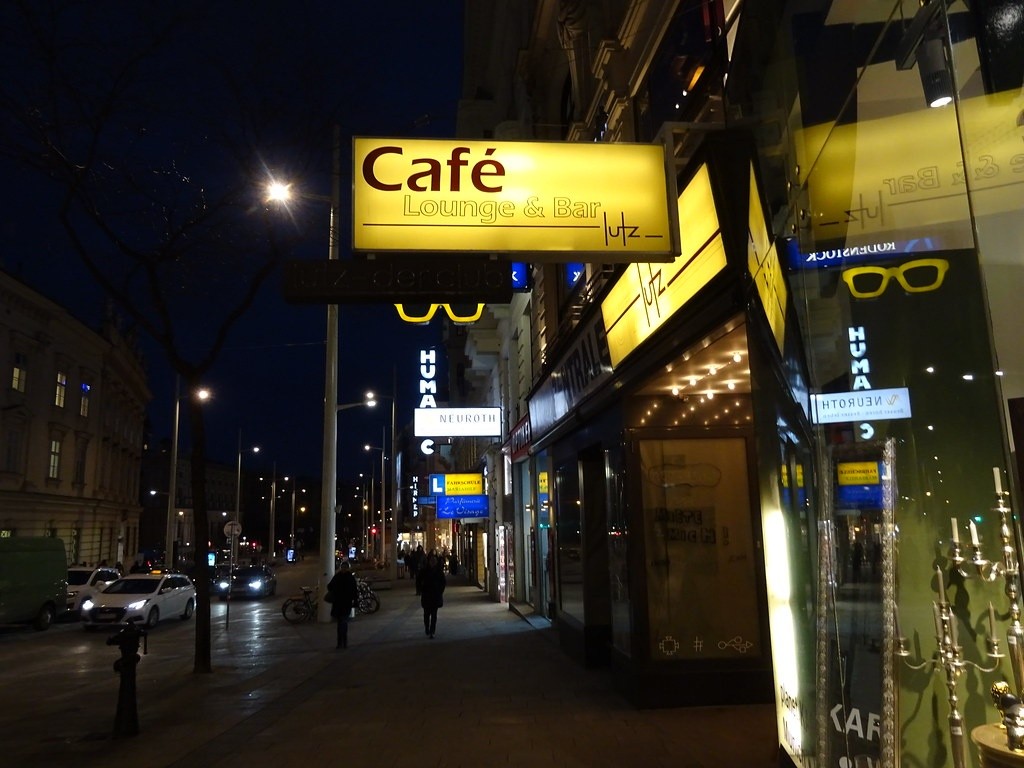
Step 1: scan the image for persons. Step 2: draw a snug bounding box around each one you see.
[322,562,361,650]
[416,555,446,639]
[435,549,458,576]
[397,545,436,580]
[131,559,149,573]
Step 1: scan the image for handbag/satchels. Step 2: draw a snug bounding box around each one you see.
[324,591,333,604]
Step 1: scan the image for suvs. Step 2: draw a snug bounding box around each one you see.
[0,536,122,631]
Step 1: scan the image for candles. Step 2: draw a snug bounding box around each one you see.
[890,463,1014,649]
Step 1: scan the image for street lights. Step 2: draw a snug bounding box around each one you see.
[355,469,374,559]
[291,476,306,550]
[365,425,386,568]
[164,377,210,570]
[365,370,399,591]
[234,429,260,564]
[260,461,290,559]
[317,303,379,623]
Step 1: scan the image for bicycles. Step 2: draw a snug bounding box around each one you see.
[282,567,381,625]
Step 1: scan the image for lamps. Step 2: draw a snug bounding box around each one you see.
[917,39,954,108]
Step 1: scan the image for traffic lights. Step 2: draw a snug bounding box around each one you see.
[372,523,376,532]
[252,541,257,551]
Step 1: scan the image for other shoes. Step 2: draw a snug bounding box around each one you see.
[429,634,435,639]
[425,628,430,635]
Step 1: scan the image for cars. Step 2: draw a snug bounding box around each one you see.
[80,574,196,630]
[210,563,276,601]
